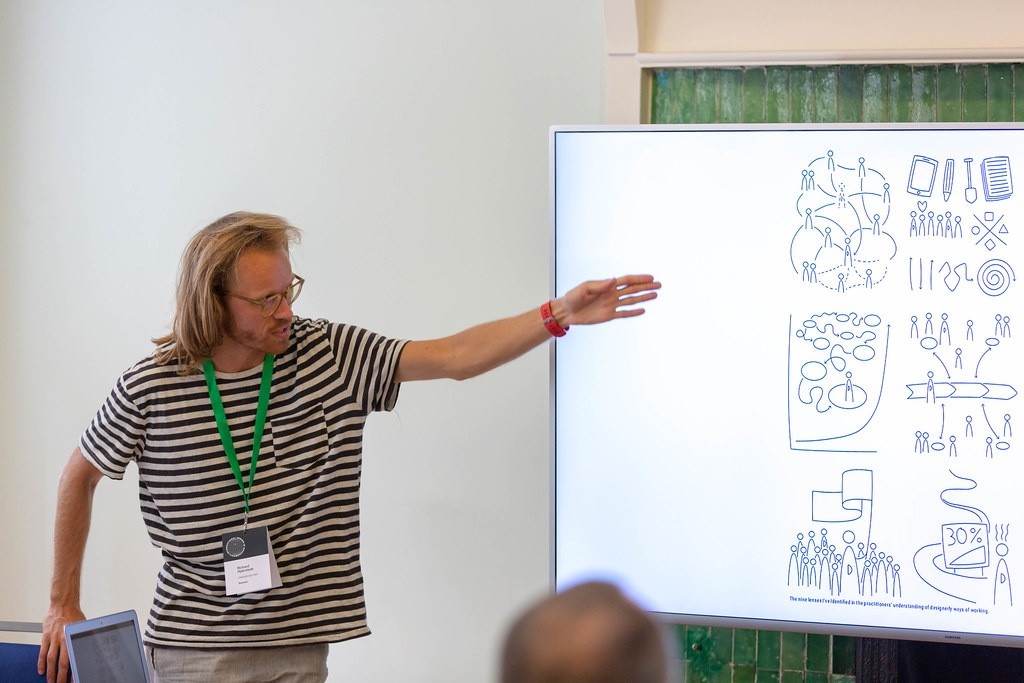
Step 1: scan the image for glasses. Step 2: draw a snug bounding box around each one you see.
[224,273,305,317]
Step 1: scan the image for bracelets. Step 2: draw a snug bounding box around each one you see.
[540,300,570,337]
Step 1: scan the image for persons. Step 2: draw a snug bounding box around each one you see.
[38,210,662,683]
[499,580,670,683]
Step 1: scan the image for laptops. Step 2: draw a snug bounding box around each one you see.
[64,609,150,683]
